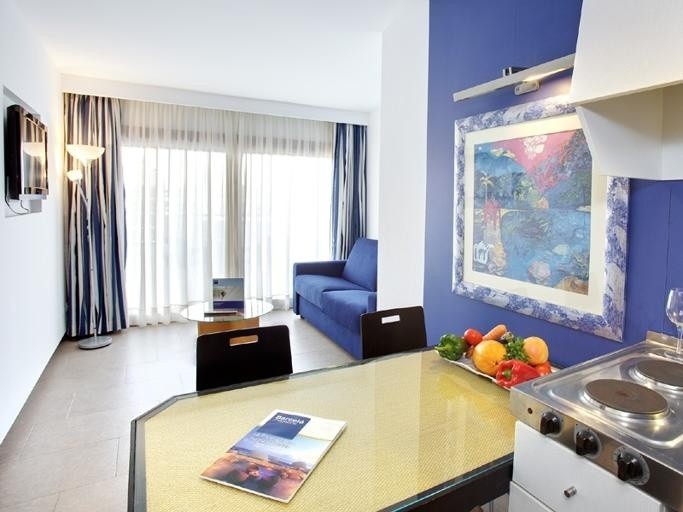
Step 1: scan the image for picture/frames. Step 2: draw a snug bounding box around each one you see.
[448,92,630,343]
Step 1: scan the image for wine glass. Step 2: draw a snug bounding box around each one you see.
[663,287,683,361]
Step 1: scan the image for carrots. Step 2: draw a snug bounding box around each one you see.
[483,324,506,341]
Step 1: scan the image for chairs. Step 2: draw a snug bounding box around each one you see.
[356,304,425,361]
[189,326,294,391]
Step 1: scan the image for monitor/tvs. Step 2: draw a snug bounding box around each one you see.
[6,105,49,201]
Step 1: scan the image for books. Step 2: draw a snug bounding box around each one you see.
[212,277,245,310]
[200,408,348,504]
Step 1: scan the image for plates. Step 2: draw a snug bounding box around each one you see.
[436,346,560,390]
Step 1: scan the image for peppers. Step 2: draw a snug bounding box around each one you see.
[437,332,465,360]
[495,360,541,388]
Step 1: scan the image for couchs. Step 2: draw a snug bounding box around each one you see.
[290,234,377,361]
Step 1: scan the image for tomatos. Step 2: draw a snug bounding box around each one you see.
[536,364,549,375]
[464,327,482,347]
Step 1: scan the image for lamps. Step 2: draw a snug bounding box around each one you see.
[60,141,113,352]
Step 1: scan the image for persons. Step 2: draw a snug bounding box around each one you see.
[257,472,289,495]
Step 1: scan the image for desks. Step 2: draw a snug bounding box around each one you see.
[120,342,511,512]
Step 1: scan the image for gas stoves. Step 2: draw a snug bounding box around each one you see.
[507,342,683,512]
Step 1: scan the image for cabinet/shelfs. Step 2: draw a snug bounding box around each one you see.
[506,417,664,512]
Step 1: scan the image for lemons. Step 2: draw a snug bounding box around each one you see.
[523,335,549,366]
[472,339,507,376]
